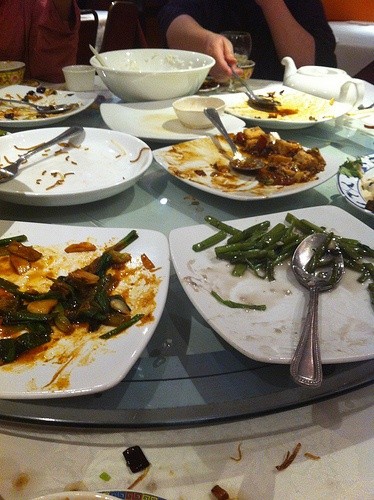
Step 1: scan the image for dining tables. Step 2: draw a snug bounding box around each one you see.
[0,74,374,500]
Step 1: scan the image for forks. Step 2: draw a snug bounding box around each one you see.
[0,125,82,185]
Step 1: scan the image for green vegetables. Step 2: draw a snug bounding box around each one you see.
[0,228,145,365]
[338,158,363,178]
[192,214,374,314]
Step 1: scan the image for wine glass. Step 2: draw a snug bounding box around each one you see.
[217,30,253,94]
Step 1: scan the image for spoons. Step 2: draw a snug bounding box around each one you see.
[289,233,344,387]
[231,71,281,108]
[202,106,266,172]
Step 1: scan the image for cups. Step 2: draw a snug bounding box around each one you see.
[62,65,96,93]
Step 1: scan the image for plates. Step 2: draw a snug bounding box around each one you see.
[198,84,221,93]
[99,98,245,142]
[0,84,99,128]
[0,217,170,400]
[168,205,373,362]
[151,134,353,198]
[337,151,374,216]
[0,125,150,207]
[209,83,355,133]
[353,114,374,136]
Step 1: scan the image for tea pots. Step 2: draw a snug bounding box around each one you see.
[281,56,364,107]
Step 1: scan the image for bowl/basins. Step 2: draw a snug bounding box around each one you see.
[173,95,226,129]
[0,61,26,87]
[212,59,256,83]
[89,48,214,101]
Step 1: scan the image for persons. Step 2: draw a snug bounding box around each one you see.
[0,0,80,83]
[137,0,337,83]
